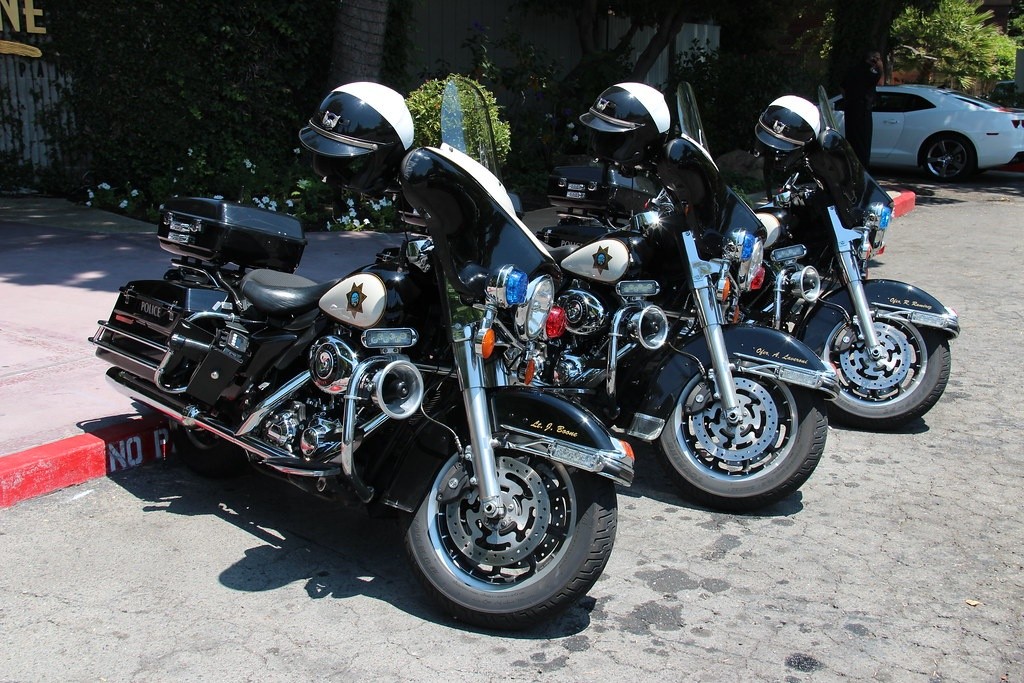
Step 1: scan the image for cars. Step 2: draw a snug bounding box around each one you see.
[816,83,1024,188]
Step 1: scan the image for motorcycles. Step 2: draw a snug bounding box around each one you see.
[78,80,635,624]
[536,77,842,506]
[752,95,961,425]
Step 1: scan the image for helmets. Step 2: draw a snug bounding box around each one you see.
[755,95,820,176]
[579,82,670,178]
[298,82,414,192]
